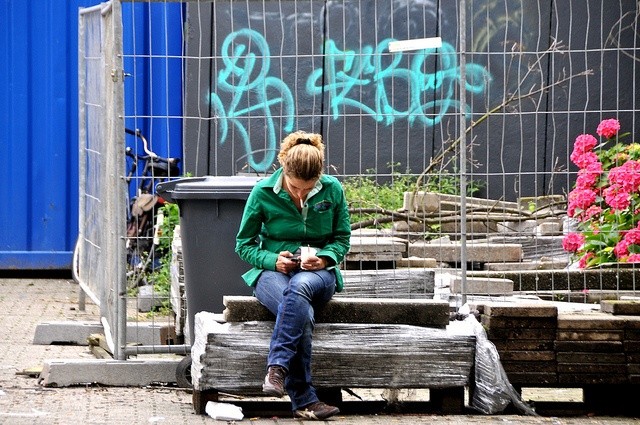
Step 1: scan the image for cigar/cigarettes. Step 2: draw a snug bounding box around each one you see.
[300,198,304,208]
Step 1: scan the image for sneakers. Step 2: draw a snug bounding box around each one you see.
[262,366,285,400]
[293,401,340,420]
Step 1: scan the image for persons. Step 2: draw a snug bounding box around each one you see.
[235,129,351,421]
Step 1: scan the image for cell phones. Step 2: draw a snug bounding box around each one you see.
[287,252,303,269]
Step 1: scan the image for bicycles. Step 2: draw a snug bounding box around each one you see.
[72,147,163,295]
[125,127,180,279]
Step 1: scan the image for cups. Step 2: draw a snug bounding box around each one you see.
[300,245,318,272]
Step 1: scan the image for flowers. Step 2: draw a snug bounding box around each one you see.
[562,117,640,269]
